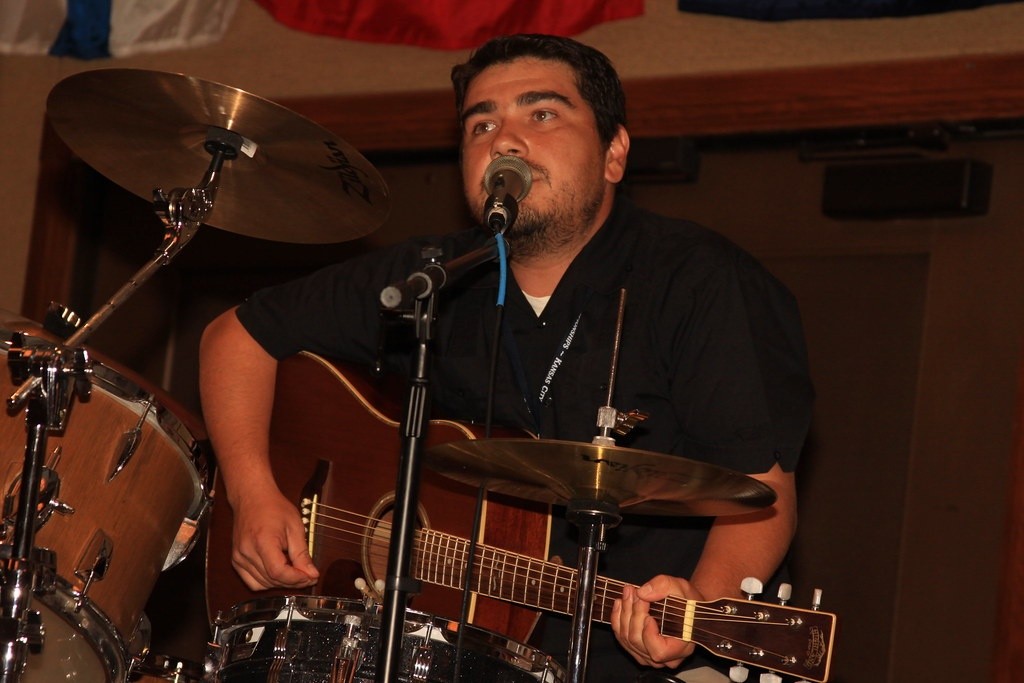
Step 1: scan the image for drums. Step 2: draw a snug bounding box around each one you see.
[0,333,207,683]
[213,593,566,683]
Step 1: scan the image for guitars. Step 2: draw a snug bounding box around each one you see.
[206,350,838,683]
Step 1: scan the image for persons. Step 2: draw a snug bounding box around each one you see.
[199,34,815,683]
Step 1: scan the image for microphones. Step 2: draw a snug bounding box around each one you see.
[483,155,532,230]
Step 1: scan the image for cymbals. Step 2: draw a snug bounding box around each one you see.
[45,67,393,244]
[421,439,777,516]
